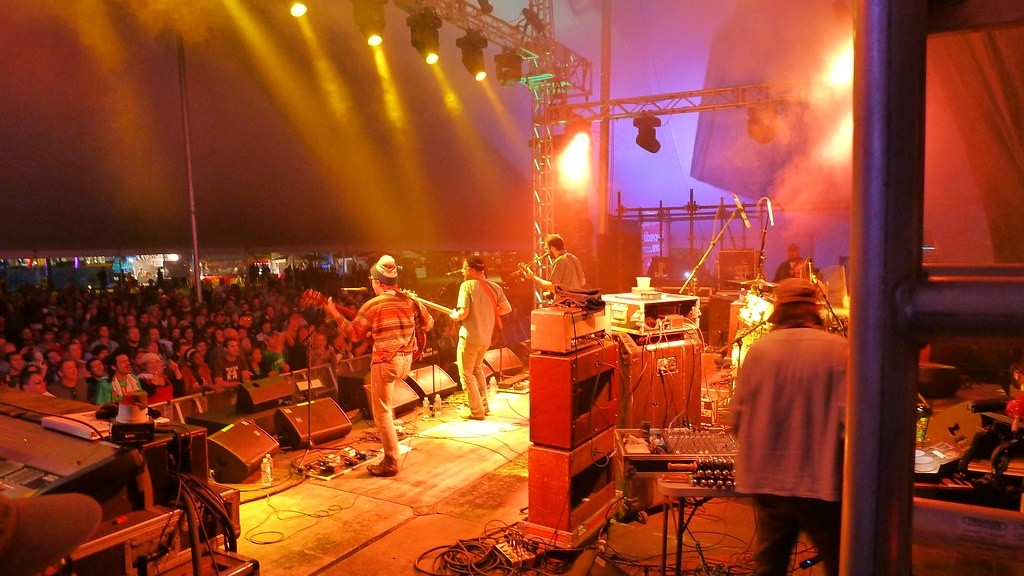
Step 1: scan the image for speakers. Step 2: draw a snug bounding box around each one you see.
[360,379,419,421]
[273,397,352,450]
[236,375,294,414]
[527,306,619,530]
[405,364,458,405]
[446,360,493,392]
[206,418,277,483]
[519,339,539,364]
[481,347,523,380]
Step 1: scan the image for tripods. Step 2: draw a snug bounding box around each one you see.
[280,293,358,466]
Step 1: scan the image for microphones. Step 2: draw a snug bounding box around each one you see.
[446,270,462,276]
[732,194,751,228]
[535,253,548,263]
[766,198,775,226]
[341,287,368,294]
[808,257,813,283]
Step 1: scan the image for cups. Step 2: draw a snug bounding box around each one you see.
[636,276,651,288]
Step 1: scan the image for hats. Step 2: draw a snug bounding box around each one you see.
[370,255,397,285]
[467,255,484,271]
[774,277,817,304]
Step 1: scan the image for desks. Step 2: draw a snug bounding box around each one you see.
[657,474,974,576]
[968,459,1024,477]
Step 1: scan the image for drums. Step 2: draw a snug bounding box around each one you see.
[736,290,776,331]
[730,328,768,391]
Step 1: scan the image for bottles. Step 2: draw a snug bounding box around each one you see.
[489,375,497,397]
[265,454,274,481]
[423,397,430,418]
[435,394,442,415]
[261,458,271,485]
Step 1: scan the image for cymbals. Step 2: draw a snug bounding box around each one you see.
[728,279,779,287]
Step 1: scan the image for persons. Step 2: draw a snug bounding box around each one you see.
[526,235,586,306]
[249,264,271,283]
[449,255,512,418]
[284,263,337,282]
[119,267,145,292]
[98,266,108,294]
[0,281,467,407]
[771,244,823,293]
[0,491,103,576]
[324,255,434,477]
[157,268,164,285]
[730,278,848,576]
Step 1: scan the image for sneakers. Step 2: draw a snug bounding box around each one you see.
[460,412,485,420]
[366,454,399,476]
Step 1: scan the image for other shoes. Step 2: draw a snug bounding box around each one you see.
[972,473,1002,485]
[944,470,968,479]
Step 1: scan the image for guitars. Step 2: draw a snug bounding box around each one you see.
[517,261,555,305]
[397,287,500,347]
[302,288,428,364]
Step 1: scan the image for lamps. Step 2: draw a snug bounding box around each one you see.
[747,107,775,144]
[493,44,523,86]
[283,0,308,17]
[456,27,487,81]
[349,0,388,47]
[633,109,661,153]
[406,0,442,65]
